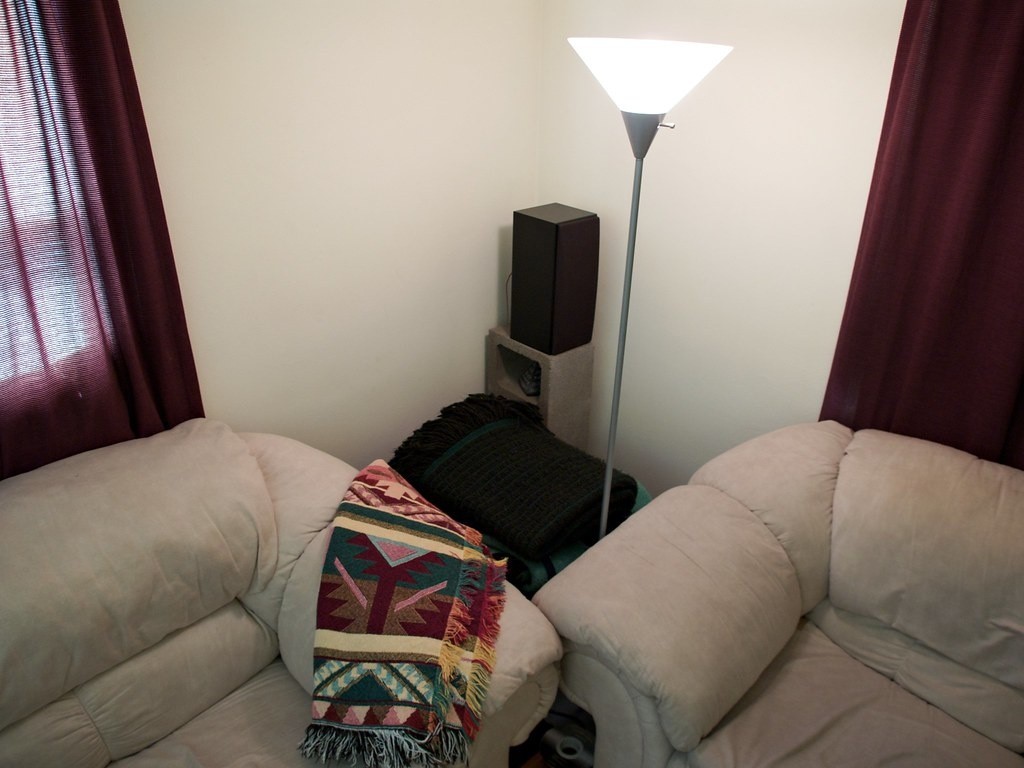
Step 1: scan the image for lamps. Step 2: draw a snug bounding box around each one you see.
[568,36,733,540]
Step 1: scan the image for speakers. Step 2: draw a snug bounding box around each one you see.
[511,202,600,357]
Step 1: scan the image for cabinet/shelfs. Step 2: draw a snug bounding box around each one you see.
[485,321,595,452]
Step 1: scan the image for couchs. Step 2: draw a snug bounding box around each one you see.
[533,420,1023,768]
[0,418,566,768]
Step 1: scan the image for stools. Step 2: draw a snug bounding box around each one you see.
[386,393,653,601]
